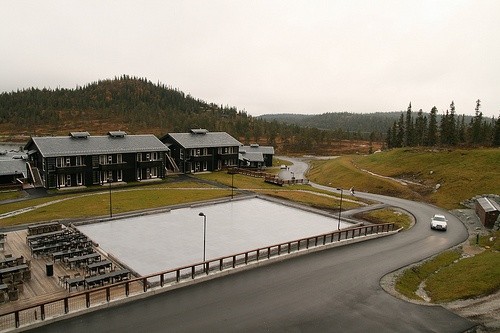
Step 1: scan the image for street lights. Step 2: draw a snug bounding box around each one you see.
[336,187,343,231]
[198,212,207,274]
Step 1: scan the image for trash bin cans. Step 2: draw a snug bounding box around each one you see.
[45,263,53,276]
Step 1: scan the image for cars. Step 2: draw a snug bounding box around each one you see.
[430,214,448,231]
[280,164,286,169]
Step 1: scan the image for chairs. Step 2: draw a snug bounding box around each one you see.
[0,221,131,304]
